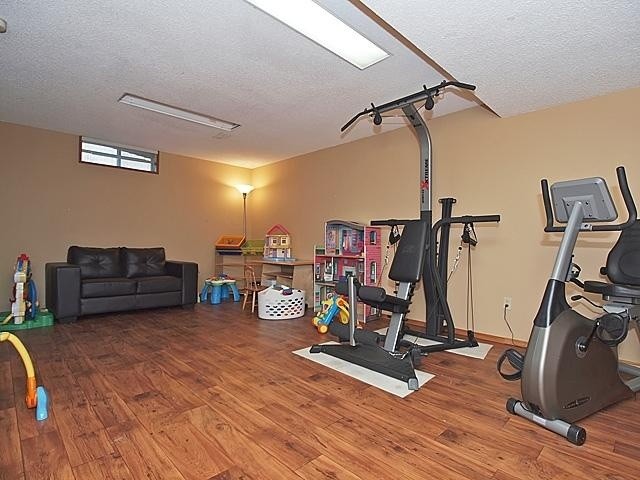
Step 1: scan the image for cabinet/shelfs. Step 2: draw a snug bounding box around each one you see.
[215,249,314,309]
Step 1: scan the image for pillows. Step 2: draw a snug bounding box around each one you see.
[67,246,123,278]
[121,247,168,279]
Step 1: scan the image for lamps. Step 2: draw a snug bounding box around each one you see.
[235,185,254,236]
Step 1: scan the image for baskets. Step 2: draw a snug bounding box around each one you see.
[258,283,305,320]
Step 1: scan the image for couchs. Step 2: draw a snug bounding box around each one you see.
[45,259,198,323]
